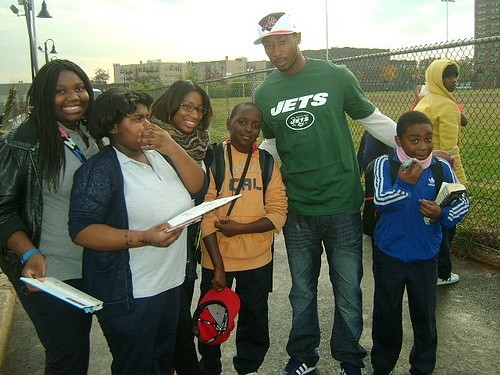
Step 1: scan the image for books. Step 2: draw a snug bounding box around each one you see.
[424,182,467,226]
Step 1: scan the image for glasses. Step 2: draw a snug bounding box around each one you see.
[180,103,207,115]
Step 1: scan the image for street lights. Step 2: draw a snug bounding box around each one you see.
[10,0,52,79]
[38,39,57,64]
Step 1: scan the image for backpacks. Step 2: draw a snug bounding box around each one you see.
[363,153,442,237]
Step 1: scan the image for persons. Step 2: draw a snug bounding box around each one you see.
[251,12,456,375]
[148,80,213,375]
[367,112,469,374]
[69,87,210,375]
[196,102,289,375]
[0,59,109,375]
[412,59,469,285]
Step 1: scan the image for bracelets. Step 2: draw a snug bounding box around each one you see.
[19,248,47,264]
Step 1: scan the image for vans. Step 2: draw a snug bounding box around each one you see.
[92,87,101,98]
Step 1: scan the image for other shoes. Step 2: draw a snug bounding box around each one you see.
[245,372,260,375]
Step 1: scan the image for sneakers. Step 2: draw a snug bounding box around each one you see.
[340,363,362,375]
[436,271,459,285]
[282,358,316,375]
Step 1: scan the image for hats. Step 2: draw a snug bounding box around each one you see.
[192,287,241,345]
[254,12,299,45]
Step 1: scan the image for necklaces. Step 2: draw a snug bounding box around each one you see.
[225,141,254,220]
